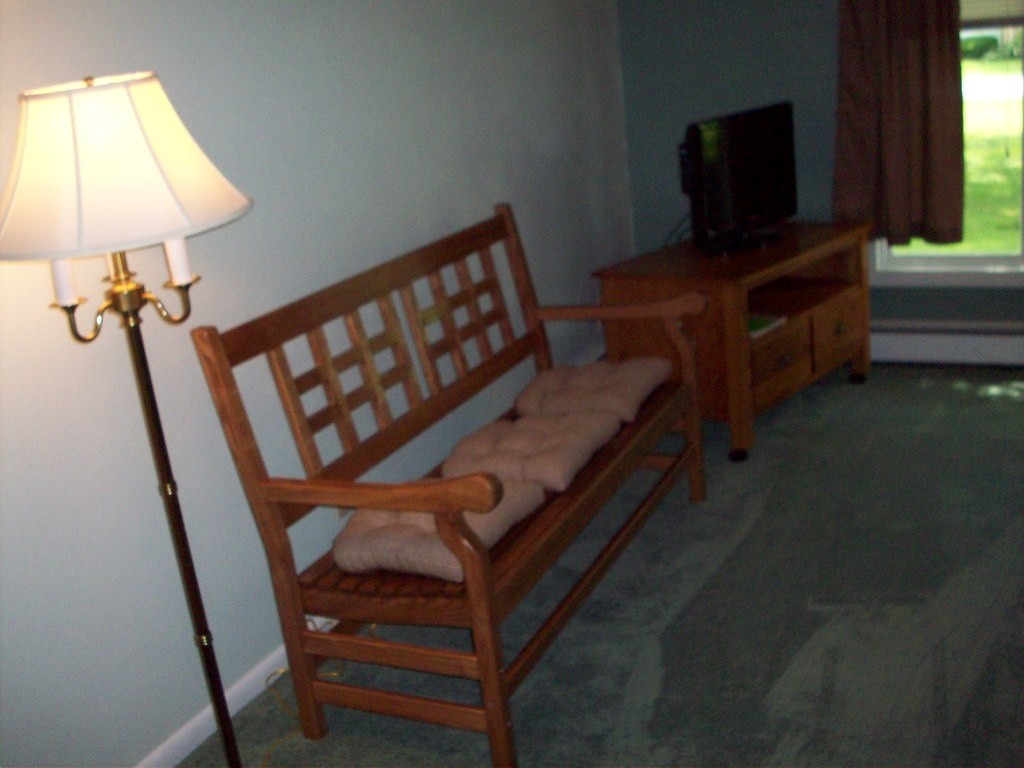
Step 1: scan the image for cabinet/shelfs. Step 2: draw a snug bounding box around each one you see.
[593,221,877,463]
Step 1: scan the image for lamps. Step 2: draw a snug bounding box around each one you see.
[1,71,252,767]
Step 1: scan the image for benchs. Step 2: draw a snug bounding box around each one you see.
[191,204,709,768]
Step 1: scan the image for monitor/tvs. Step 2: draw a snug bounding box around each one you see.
[681,103,798,255]
